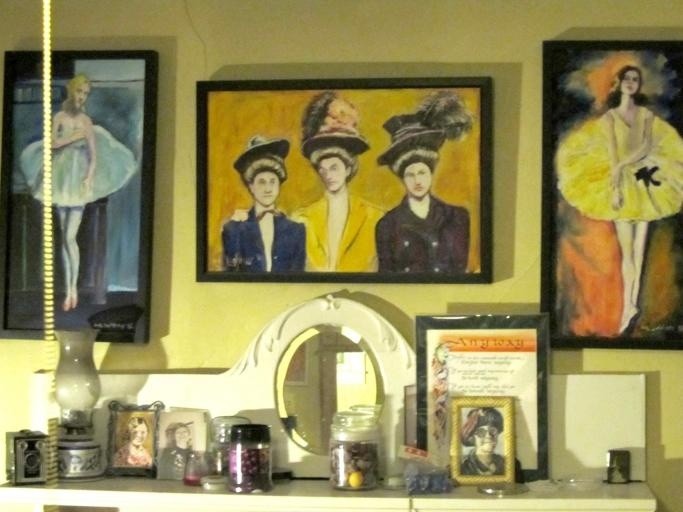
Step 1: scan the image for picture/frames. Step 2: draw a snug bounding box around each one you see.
[541,36,683,351]
[0,49,160,348]
[414,311,549,480]
[154,407,209,484]
[451,395,516,486]
[103,399,164,479]
[193,78,494,285]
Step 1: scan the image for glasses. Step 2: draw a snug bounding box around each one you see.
[474,426,497,438]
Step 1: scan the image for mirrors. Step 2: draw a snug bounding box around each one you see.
[269,320,388,460]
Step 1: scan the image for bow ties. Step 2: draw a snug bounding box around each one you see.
[256,210,281,222]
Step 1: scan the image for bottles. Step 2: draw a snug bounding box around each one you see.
[230,424,273,494]
[329,410,380,491]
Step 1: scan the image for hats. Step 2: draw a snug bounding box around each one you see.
[377,90,472,166]
[234,137,290,173]
[302,92,371,159]
[461,408,503,446]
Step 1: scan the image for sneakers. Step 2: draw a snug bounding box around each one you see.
[69,294,77,308]
[63,297,71,311]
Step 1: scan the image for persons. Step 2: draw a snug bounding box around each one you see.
[555,65,683,334]
[290,92,388,271]
[221,134,306,272]
[112,417,152,468]
[19,76,136,310]
[375,91,470,272]
[459,406,505,476]
